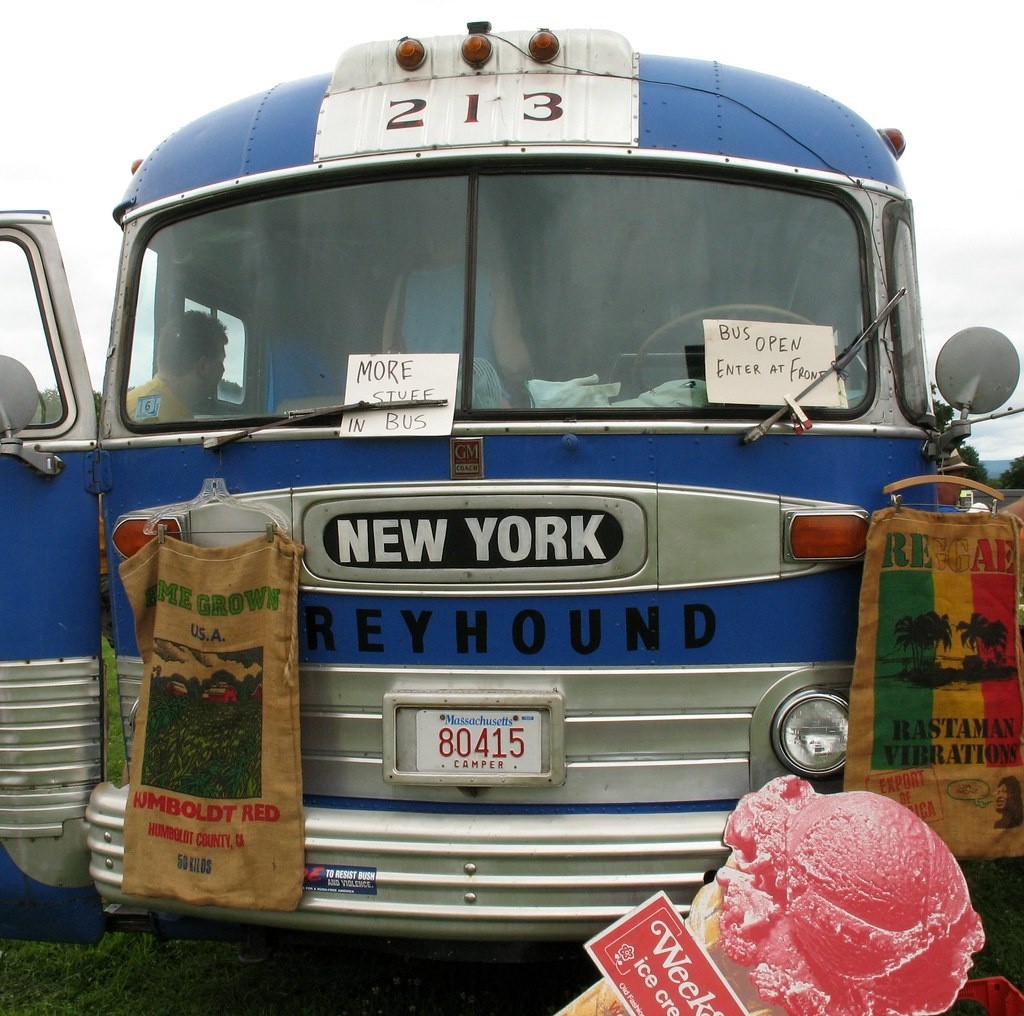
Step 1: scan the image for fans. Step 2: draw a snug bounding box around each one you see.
[455,361,504,412]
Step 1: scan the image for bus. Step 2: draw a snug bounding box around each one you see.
[0,13,1024,958]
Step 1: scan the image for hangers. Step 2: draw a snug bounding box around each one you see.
[882,432,1004,519]
[143,445,291,543]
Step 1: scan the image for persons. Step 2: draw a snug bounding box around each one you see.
[125,311,229,425]
[936,448,1024,522]
[381,192,546,388]
